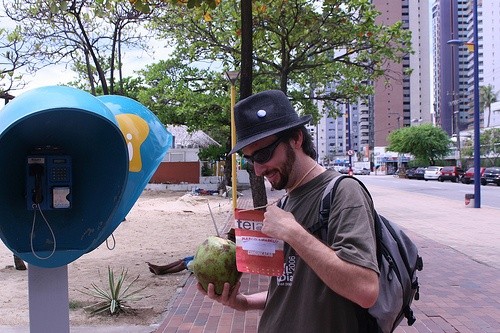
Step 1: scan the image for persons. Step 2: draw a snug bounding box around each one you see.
[147,228,236,275]
[196,90,380,333]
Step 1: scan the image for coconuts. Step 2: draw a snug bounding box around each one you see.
[193,235,244,294]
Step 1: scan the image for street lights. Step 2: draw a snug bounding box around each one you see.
[446,39,482,209]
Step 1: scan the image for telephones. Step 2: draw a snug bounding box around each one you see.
[25,154,73,209]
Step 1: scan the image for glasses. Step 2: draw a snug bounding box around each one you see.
[245,128,293,166]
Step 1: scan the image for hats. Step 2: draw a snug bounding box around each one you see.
[227,90,312,157]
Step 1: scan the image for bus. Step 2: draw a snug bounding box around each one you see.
[320,163,350,173]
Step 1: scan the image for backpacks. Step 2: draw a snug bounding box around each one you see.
[277,174,423,333]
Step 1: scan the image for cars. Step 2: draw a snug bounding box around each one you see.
[480,167,500,186]
[438,166,464,183]
[424,166,445,181]
[353,167,370,175]
[406,168,416,179]
[461,167,488,186]
[413,167,427,180]
